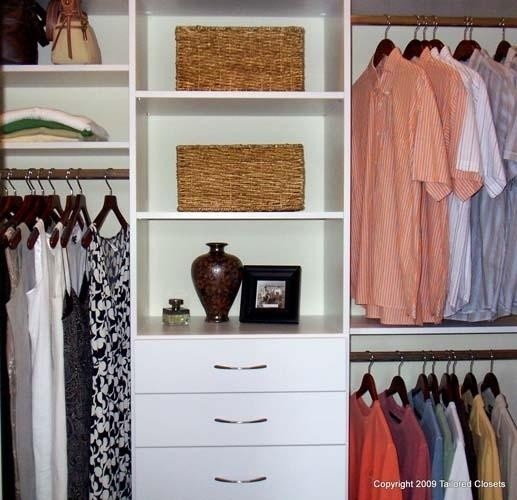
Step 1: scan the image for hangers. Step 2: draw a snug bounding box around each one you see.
[1,168,127,249]
[372,13,515,70]
[354,349,504,425]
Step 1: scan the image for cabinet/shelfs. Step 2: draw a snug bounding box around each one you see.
[348,0,516,499]
[0,0,132,499]
[132,0,347,500]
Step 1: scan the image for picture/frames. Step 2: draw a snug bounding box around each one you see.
[239,264,301,325]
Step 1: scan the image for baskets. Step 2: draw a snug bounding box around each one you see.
[175,144,307,213]
[175,25,306,91]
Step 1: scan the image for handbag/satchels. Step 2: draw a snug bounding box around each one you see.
[45,0,102,65]
[1,0,48,65]
